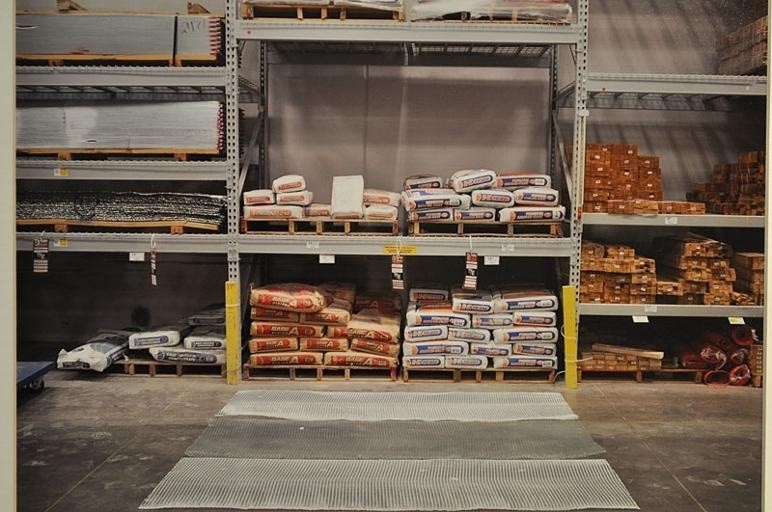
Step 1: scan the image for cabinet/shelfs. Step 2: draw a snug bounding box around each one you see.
[573,0,771,388]
[14,0,231,385]
[230,0,584,387]
